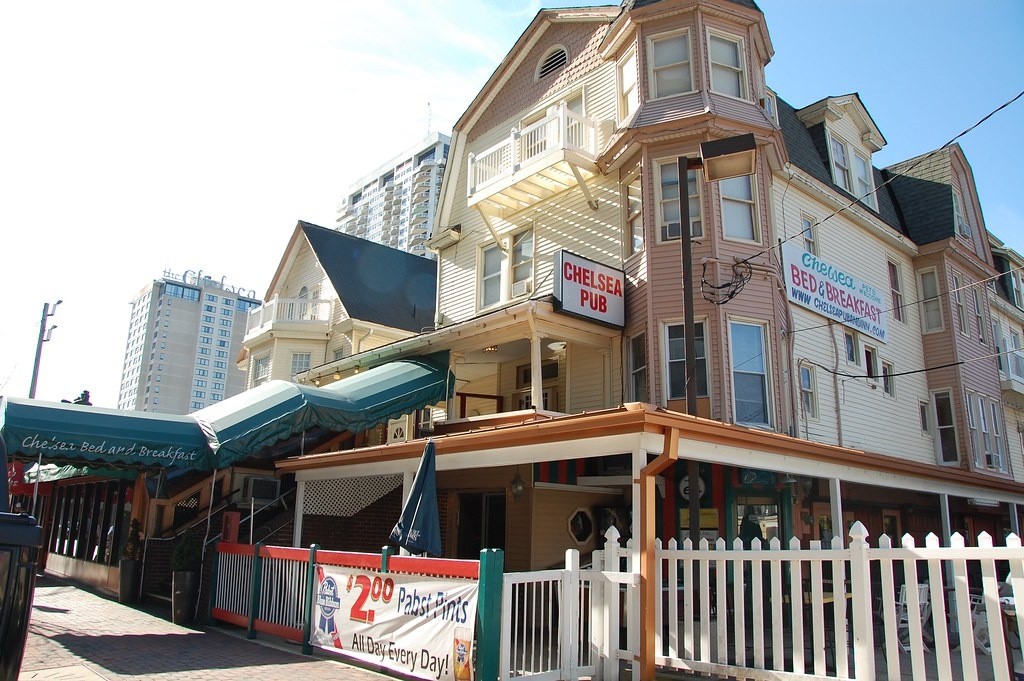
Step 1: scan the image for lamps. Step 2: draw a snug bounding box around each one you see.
[510,466,530,499]
[967,499,999,507]
[482,347,497,353]
[354,366,360,373]
[315,377,320,386]
[778,474,796,483]
[61,390,92,405]
[333,367,341,380]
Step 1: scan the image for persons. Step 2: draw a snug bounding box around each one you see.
[16,501,25,514]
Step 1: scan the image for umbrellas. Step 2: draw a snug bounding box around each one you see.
[390,438,441,557]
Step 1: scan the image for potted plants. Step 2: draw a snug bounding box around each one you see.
[171,527,201,624]
[119,517,143,604]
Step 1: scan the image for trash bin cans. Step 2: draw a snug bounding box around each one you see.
[171,570,198,625]
[118,559,138,603]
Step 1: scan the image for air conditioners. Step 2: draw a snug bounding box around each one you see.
[960,223,972,238]
[986,454,1002,469]
[242,477,281,507]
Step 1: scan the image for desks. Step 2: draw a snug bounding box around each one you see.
[770,591,853,671]
[555,579,698,650]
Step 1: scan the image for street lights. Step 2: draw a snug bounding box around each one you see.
[677,131,758,618]
[29,300,63,397]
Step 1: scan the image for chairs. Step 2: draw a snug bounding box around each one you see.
[873,583,933,655]
[948,586,991,655]
